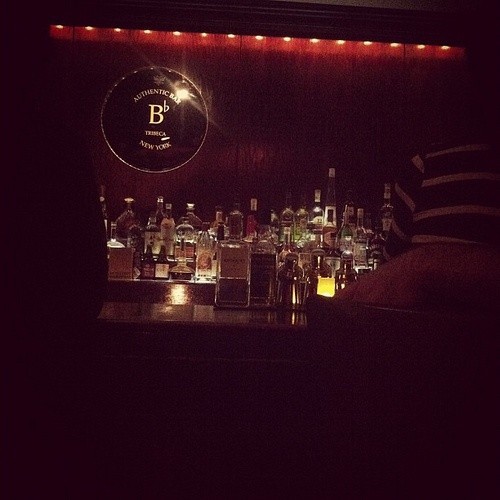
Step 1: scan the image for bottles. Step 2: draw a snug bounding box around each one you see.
[100,166,391,311]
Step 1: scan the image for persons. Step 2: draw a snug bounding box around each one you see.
[331,242,500,305]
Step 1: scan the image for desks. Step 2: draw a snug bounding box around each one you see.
[97,279,308,500]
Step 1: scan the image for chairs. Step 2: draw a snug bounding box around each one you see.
[302,292,500,500]
